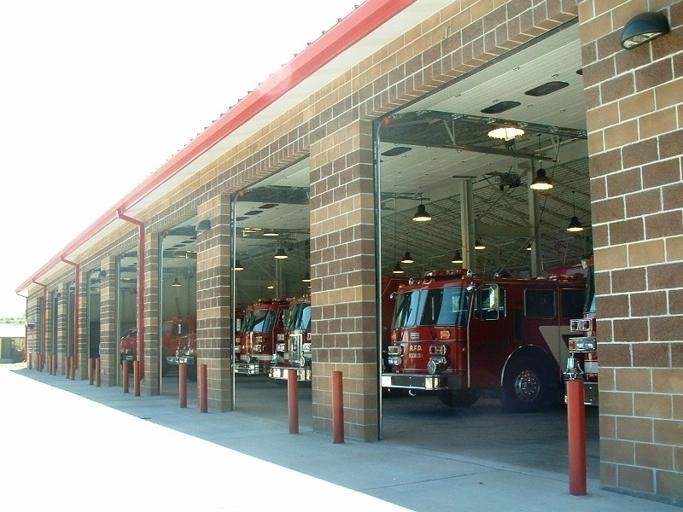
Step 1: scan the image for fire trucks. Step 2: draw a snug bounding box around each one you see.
[376,254,593,407]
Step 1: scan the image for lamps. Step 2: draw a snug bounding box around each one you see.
[273,248,288,260]
[230,259,244,272]
[390,194,487,274]
[530,132,585,233]
[619,12,671,51]
[193,219,212,233]
